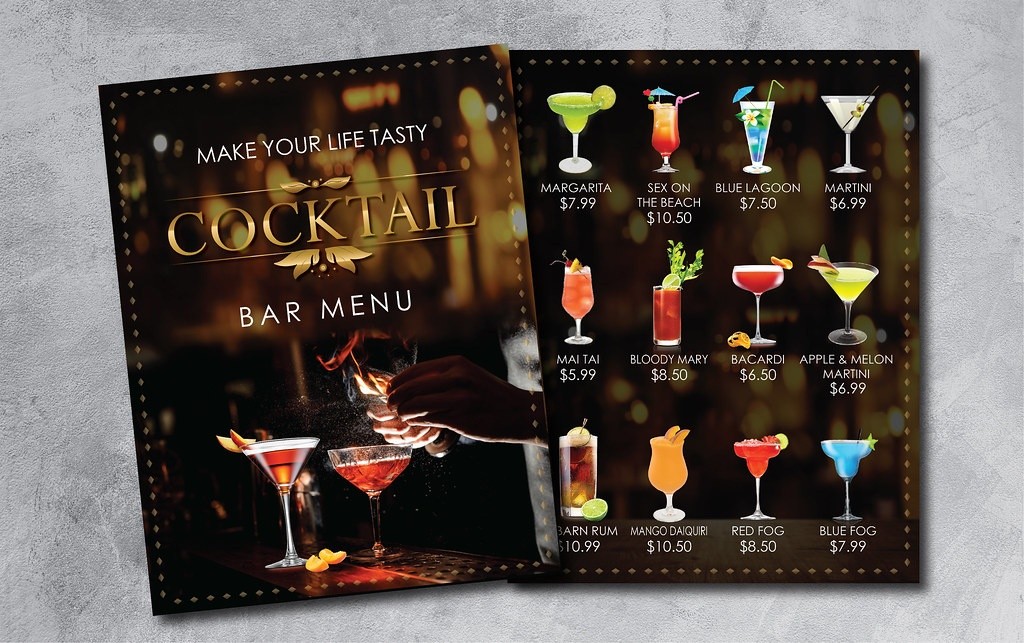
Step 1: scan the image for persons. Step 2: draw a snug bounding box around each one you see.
[367,356,546,458]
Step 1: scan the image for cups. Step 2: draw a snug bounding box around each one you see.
[739,101,775,174]
[559,436,598,519]
[654,287,682,346]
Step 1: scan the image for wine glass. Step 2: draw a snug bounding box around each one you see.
[650,104,681,172]
[733,442,780,519]
[561,266,595,345]
[818,262,879,346]
[547,91,604,174]
[732,265,784,347]
[648,436,689,523]
[821,96,876,174]
[822,439,874,521]
[240,437,321,568]
[328,444,412,560]
